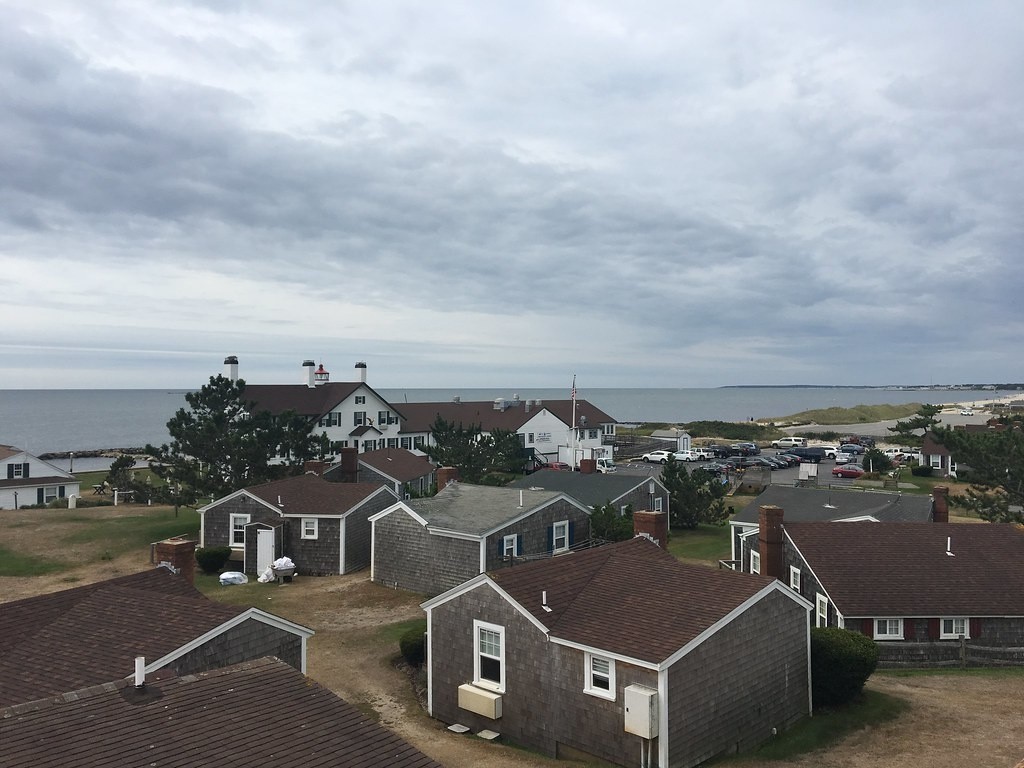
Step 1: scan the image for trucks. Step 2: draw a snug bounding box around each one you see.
[839,432,860,446]
[575,457,617,474]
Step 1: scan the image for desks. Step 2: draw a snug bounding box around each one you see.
[92,485,106,495]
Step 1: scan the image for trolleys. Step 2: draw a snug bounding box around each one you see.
[269,564,297,583]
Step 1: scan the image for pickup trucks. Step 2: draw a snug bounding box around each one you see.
[883,447,919,461]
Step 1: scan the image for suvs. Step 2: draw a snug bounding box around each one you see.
[776,447,826,463]
[771,436,808,449]
[858,435,875,448]
[689,448,715,461]
[641,450,674,465]
[738,443,761,455]
[730,445,749,457]
[707,444,732,458]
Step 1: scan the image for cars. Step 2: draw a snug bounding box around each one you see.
[691,454,803,478]
[823,445,837,459]
[961,410,973,416]
[673,450,699,461]
[831,464,866,478]
[534,461,572,472]
[837,443,866,455]
[835,453,857,465]
